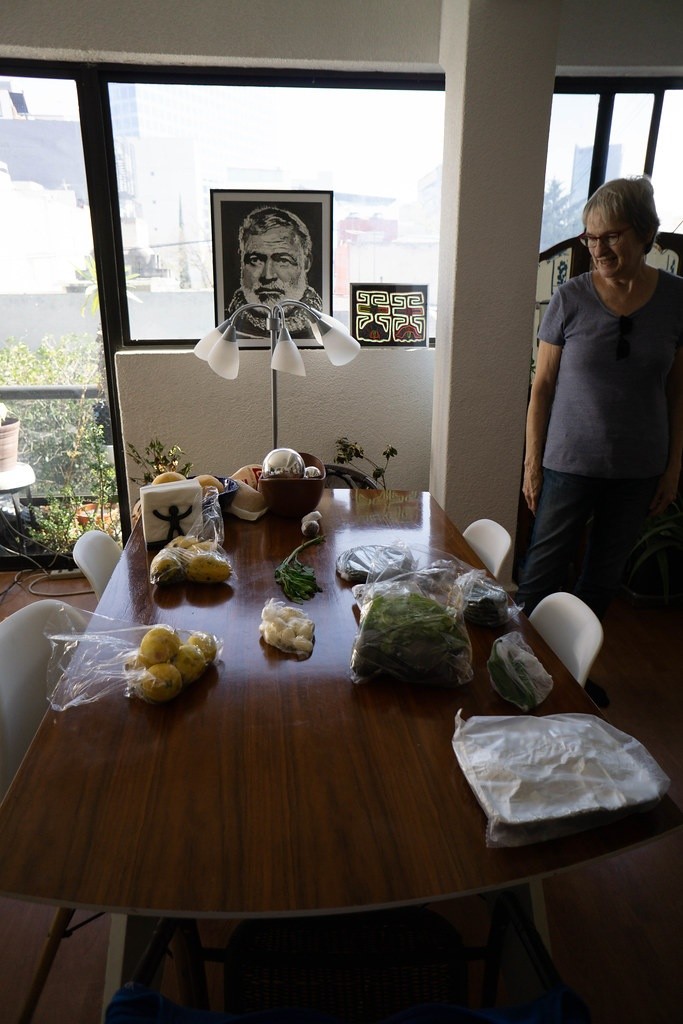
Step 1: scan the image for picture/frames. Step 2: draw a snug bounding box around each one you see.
[210,187,332,350]
[350,283,429,348]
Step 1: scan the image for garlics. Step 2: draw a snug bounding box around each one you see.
[259,604,314,654]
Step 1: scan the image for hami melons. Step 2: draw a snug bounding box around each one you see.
[151,472,186,485]
[194,474,224,496]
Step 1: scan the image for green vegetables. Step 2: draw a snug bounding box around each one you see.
[349,593,536,709]
[274,534,325,605]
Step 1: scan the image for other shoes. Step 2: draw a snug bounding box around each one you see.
[585,679,610,708]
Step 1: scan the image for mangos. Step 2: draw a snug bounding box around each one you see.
[150,535,233,584]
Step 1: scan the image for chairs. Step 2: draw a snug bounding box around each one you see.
[106,892,593,1024]
[0,599,88,800]
[325,464,379,490]
[462,517,515,581]
[527,592,606,691]
[72,530,122,603]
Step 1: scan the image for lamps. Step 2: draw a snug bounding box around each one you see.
[193,299,361,448]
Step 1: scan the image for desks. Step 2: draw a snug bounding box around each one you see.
[0,462,37,555]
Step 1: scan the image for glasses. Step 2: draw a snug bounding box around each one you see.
[577,224,634,248]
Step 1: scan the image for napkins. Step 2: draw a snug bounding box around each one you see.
[140,479,203,551]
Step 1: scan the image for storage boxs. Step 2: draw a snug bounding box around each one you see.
[258,453,326,517]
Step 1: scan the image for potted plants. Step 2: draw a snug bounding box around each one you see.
[76,419,117,528]
[0,401,20,472]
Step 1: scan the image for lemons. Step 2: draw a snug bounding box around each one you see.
[133,626,217,701]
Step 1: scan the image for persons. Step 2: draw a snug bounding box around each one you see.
[224,205,323,339]
[505,177,683,711]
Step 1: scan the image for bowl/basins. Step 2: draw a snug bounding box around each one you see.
[258,452,326,519]
[183,476,240,514]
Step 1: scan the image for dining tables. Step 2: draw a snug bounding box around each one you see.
[0,485,682,1024]
